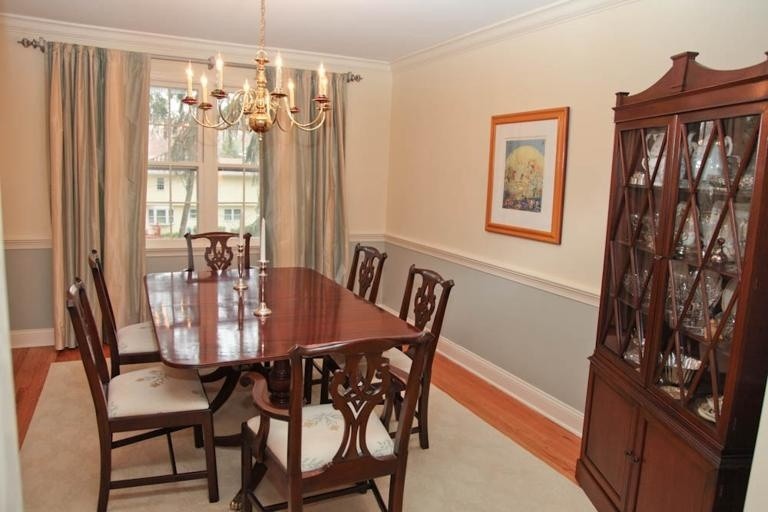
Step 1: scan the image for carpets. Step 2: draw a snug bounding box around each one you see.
[19,347,593,510]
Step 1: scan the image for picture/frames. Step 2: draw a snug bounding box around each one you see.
[485,107,569,246]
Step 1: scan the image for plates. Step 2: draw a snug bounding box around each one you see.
[720,280,744,317]
[698,404,720,424]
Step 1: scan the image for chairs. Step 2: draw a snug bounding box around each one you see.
[301,243,388,405]
[184,232,251,271]
[241,331,434,512]
[88,248,203,448]
[66,277,219,511]
[324,264,454,450]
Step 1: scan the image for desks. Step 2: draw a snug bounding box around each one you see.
[144,268,421,511]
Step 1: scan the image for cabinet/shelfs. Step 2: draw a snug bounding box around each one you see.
[572,51,768,511]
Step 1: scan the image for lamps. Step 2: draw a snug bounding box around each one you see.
[178,0,336,138]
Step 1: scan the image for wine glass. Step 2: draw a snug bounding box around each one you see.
[671,267,728,334]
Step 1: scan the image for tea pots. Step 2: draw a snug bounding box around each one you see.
[640,127,735,184]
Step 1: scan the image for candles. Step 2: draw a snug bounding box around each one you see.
[238,231,244,246]
[261,218,266,261]
